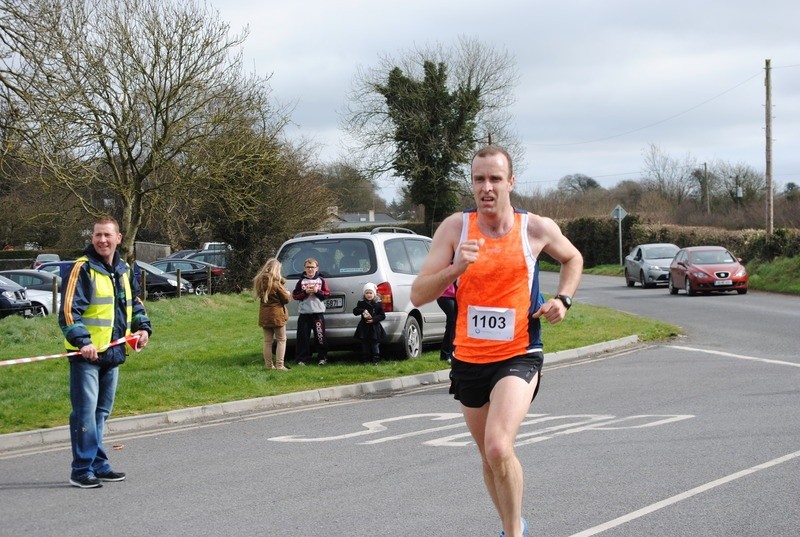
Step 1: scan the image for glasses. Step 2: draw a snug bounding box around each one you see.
[304,266,317,270]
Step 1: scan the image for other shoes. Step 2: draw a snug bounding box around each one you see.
[277,366,289,371]
[500,518,528,537]
[319,360,326,366]
[441,357,451,363]
[298,362,306,367]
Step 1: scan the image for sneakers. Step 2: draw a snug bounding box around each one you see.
[71,477,102,489]
[95,470,127,482]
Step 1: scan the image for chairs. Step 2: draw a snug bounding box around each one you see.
[339,255,360,272]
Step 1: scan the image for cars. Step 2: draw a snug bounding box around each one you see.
[0,260,86,319]
[623,242,683,289]
[668,246,749,296]
[135,241,234,302]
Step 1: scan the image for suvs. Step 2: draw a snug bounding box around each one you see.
[272,226,447,363]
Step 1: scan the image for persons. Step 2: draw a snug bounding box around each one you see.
[292,258,331,366]
[411,144,583,537]
[59,216,152,489]
[353,283,386,366]
[437,252,458,365]
[252,257,291,371]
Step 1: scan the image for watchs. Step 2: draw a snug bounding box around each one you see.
[554,294,572,310]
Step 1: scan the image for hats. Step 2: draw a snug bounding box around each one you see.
[362,283,377,294]
